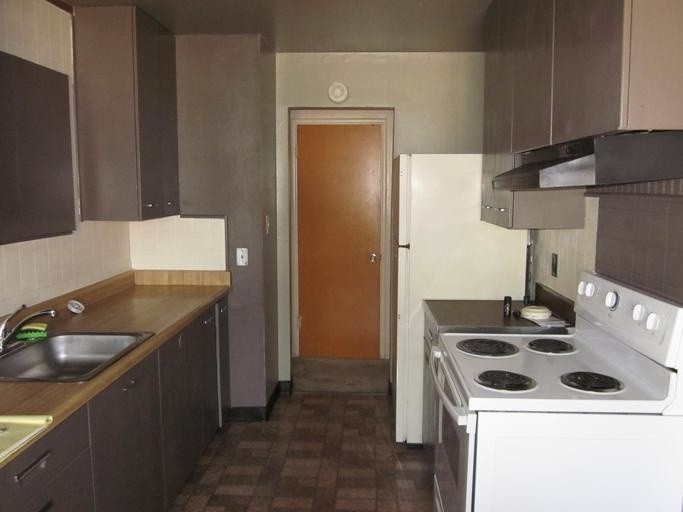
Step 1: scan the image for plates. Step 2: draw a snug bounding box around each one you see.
[521,305,552,319]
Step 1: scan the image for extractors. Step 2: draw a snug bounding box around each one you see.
[491,130,683,190]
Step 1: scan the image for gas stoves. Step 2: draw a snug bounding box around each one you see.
[438,332,662,399]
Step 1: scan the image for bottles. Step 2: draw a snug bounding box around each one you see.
[504,296,511,318]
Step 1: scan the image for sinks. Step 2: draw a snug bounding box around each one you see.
[0,334,138,379]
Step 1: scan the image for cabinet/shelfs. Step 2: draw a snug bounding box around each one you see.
[73,5,181,221]
[481,0,586,232]
[88,343,165,512]
[160,292,228,478]
[423,313,439,470]
[0,403,97,511]
[553,0,683,145]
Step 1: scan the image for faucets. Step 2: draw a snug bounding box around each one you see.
[0,304,57,350]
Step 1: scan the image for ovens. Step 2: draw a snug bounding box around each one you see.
[433,350,683,512]
